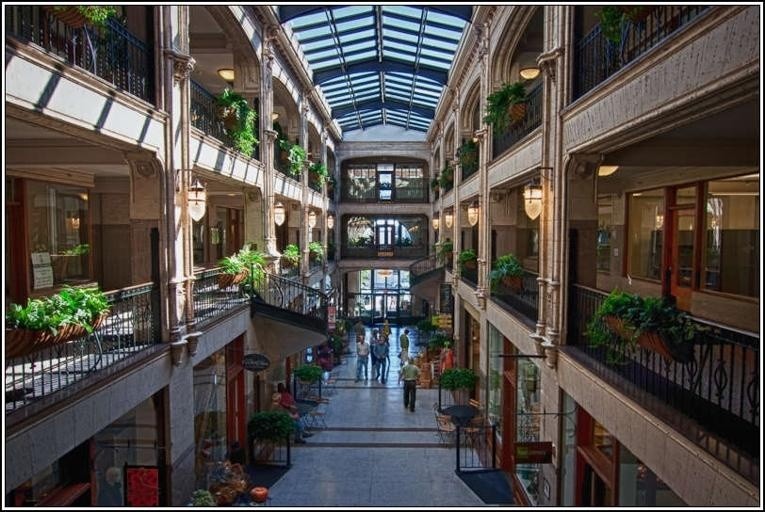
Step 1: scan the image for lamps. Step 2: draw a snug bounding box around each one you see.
[519,66,541,80]
[432,216,439,230]
[523,165,554,221]
[598,165,619,177]
[175,168,206,222]
[217,68,234,82]
[445,211,452,228]
[468,194,483,226]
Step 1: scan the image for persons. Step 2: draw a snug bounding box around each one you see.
[400,329,410,365]
[269,393,313,443]
[277,383,300,421]
[370,329,390,384]
[365,295,369,305]
[354,335,369,386]
[316,343,334,372]
[398,357,421,412]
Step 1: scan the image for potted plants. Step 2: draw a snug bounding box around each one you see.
[430,81,529,191]
[442,242,453,259]
[215,87,337,288]
[585,287,695,365]
[459,249,477,269]
[440,368,476,393]
[293,364,322,385]
[6,283,110,357]
[49,5,117,29]
[490,254,525,296]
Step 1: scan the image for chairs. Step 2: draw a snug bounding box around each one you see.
[432,399,484,452]
[326,372,339,396]
[308,398,330,430]
